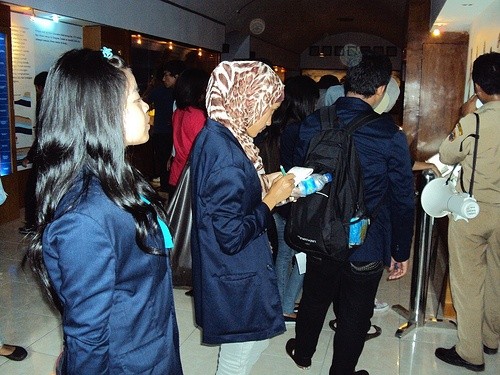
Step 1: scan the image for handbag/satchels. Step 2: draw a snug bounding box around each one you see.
[167,165,196,287]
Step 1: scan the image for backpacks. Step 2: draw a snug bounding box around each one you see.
[282,103,382,260]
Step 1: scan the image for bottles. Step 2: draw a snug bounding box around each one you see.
[276,173,335,205]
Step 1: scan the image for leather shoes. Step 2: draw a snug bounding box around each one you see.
[483,344,498,355]
[435,344,485,372]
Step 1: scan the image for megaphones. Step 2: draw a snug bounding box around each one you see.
[420,178,480,220]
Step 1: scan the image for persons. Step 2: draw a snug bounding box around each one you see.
[433,52,500,370]
[284,54,413,375]
[21,59,500,341]
[28,48,181,375]
[0,341,28,360]
[192,61,297,375]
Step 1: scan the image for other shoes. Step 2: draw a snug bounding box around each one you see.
[365,325,382,340]
[356,369,370,375]
[373,301,390,313]
[286,338,312,370]
[282,314,296,326]
[18,224,37,235]
[1,345,27,362]
[329,319,337,332]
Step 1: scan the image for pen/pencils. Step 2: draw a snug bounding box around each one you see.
[279,165,287,177]
